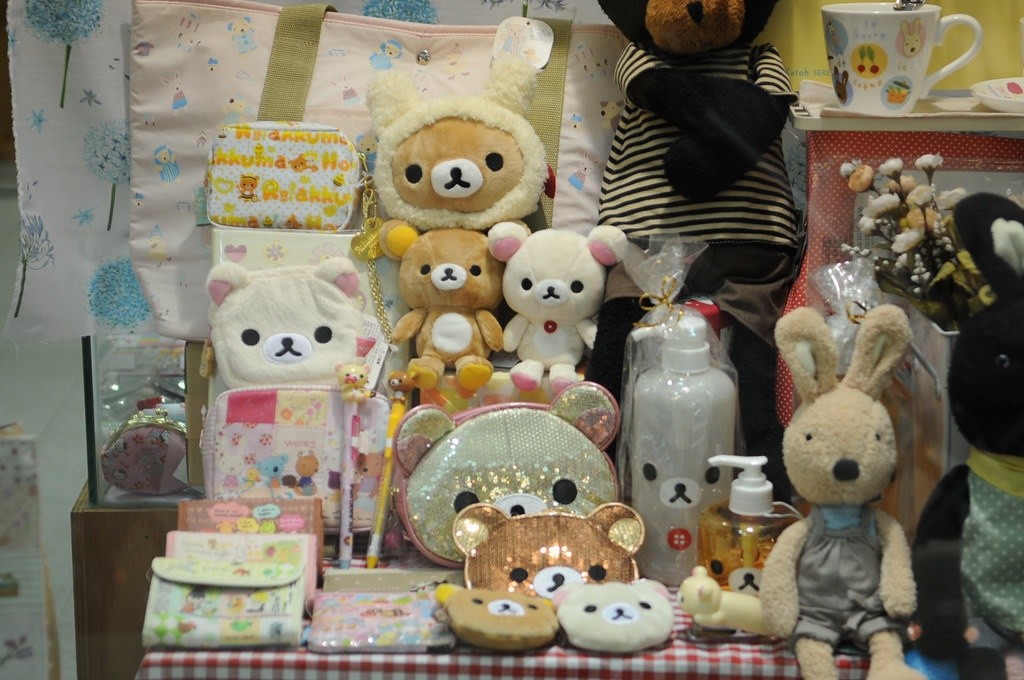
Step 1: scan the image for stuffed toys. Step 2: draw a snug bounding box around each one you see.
[487,220,629,394]
[587,0,799,504]
[366,51,548,233]
[435,581,561,653]
[203,257,363,388]
[553,578,675,655]
[913,192,1024,680]
[378,216,503,393]
[759,305,926,680]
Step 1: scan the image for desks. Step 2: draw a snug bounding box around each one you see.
[141,503,1022,676]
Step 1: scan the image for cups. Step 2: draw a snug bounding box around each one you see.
[821,3,985,116]
